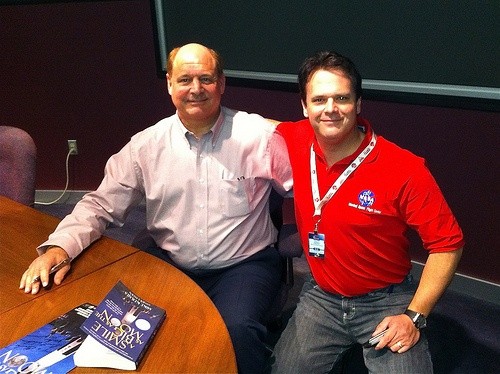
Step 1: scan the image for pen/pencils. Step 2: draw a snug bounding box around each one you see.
[30,257,73,284]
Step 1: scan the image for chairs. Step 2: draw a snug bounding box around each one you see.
[129,184,304,353]
[0,125,39,210]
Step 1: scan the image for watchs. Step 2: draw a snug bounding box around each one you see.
[404,309,428,331]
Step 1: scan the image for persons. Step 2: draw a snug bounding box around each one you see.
[21,42,296,374]
[259,52,466,374]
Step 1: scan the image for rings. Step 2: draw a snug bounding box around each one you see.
[397,341,404,348]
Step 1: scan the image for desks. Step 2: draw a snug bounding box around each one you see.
[0,196,238,373]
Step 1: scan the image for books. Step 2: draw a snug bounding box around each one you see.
[0,301,101,374]
[73,281,166,370]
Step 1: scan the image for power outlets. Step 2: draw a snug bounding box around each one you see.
[67,139,78,155]
